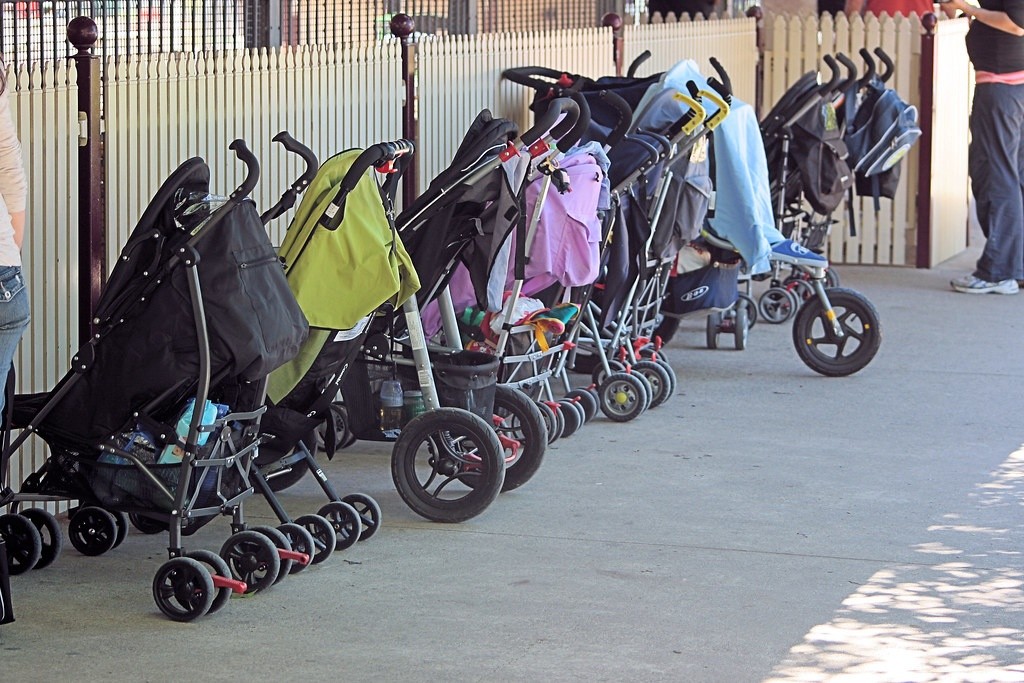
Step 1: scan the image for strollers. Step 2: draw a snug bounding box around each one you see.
[0,46,920,630]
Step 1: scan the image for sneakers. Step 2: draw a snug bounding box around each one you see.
[949,269,1019,294]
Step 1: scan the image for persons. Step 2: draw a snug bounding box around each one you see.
[935,0,1023,296]
[0,49,32,448]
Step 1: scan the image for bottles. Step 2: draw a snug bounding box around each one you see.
[156,398,217,466]
[379,380,404,438]
[404,390,425,425]
